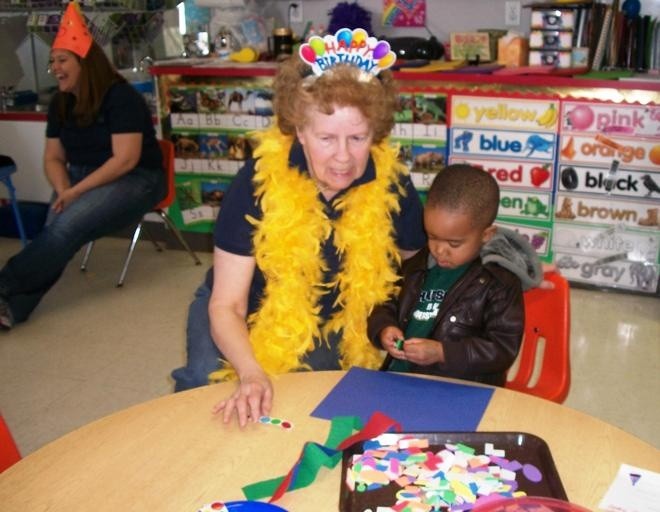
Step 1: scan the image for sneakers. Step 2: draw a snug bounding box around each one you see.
[0,297,16,330]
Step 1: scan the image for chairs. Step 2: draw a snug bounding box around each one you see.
[500,269,573,405]
[78,137,202,287]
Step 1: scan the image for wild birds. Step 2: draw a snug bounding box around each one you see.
[640,174,660,196]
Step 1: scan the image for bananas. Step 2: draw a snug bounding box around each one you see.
[536,104,558,130]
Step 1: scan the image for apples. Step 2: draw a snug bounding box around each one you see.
[569,105,594,129]
[531,164,550,187]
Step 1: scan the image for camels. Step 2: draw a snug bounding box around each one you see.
[228,91,253,112]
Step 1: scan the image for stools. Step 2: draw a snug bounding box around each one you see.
[0,153,28,250]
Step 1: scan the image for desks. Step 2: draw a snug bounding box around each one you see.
[0,368,659,512]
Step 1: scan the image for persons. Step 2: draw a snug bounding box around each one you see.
[367,163,545,387]
[167,29,426,426]
[0,37,166,333]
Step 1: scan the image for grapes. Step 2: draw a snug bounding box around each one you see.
[532,232,548,249]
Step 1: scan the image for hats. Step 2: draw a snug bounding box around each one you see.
[51,1,94,60]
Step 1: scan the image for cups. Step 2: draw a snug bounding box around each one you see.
[268,27,292,61]
